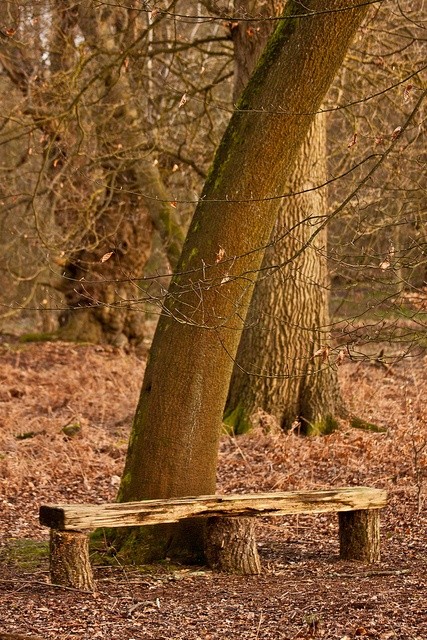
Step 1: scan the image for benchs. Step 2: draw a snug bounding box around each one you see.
[35,484,390,595]
